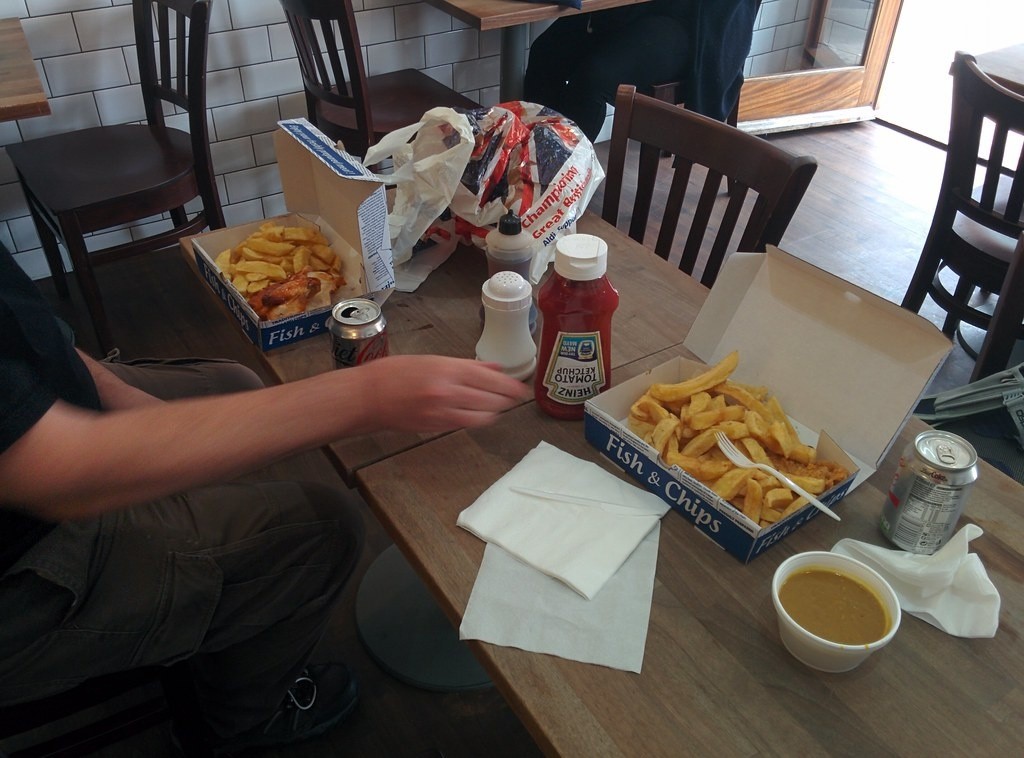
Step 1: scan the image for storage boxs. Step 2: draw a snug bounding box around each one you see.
[583,243,953,565]
[191,117,395,349]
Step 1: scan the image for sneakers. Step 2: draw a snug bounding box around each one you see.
[164,659,358,756]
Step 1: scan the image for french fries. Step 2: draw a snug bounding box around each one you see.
[627,348,826,528]
[215,221,342,295]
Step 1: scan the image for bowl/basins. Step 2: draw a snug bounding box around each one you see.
[770,549,901,673]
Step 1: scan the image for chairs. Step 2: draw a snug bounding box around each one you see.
[0,0,229,357]
[279,0,486,167]
[650,1,761,157]
[901,51,1024,386]
[600,85,817,289]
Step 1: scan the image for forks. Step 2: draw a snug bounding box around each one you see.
[715,430,843,524]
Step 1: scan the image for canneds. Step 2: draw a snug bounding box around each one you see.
[329,298,390,369]
[880,431,978,554]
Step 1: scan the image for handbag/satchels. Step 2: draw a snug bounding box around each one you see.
[361,100,606,286]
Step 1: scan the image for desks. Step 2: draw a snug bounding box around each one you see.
[949,44,1024,93]
[0,18,52,122]
[427,0,646,106]
[353,343,1024,758]
[186,213,713,491]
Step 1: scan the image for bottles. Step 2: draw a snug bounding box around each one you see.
[476,271,538,383]
[536,233,620,422]
[479,210,539,335]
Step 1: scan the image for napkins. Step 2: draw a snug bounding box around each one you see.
[458,543,662,677]
[456,436,672,597]
[834,524,1002,635]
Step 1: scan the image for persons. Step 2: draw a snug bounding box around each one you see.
[0,245,527,755]
[523,0,764,143]
